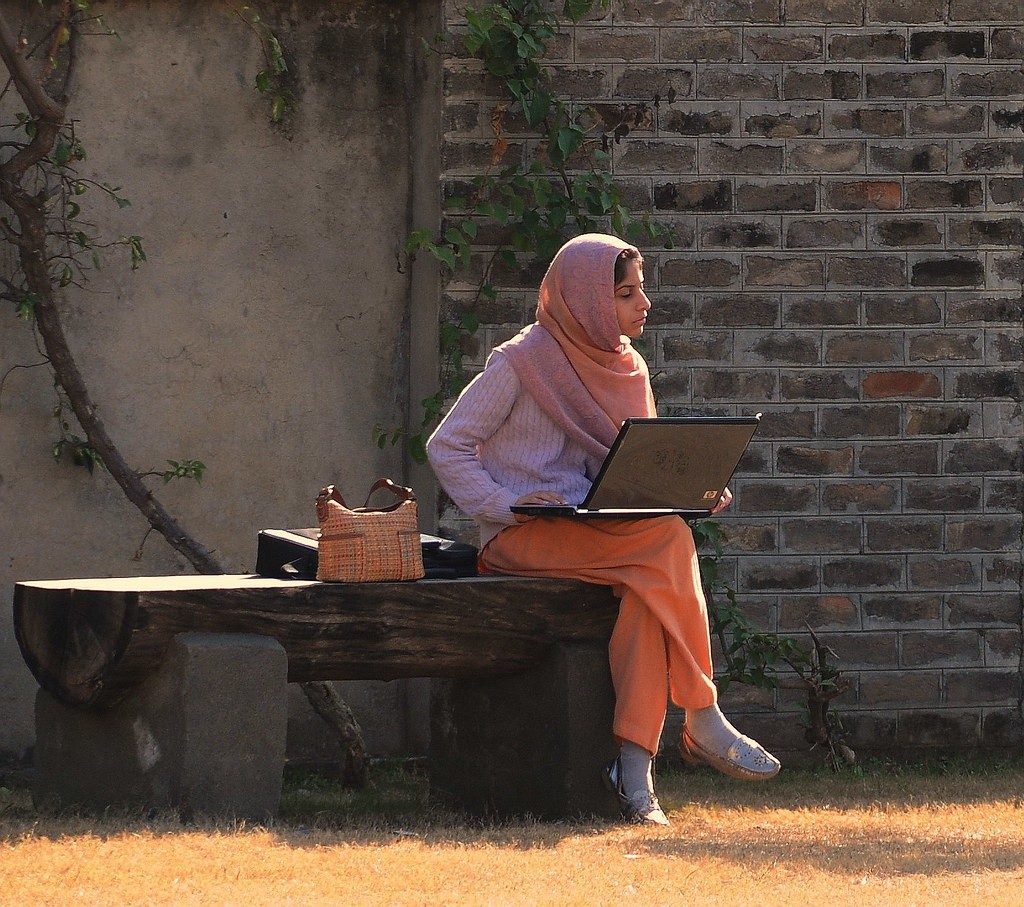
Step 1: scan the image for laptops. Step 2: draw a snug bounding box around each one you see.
[510,416,759,518]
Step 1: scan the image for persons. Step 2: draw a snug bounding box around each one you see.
[426,233,782,827]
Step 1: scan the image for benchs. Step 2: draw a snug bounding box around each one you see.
[12,574,621,820]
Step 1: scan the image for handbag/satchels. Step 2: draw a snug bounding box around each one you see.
[315,479,424,583]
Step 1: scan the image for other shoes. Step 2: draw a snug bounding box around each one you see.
[604,756,669,825]
[676,724,781,780]
